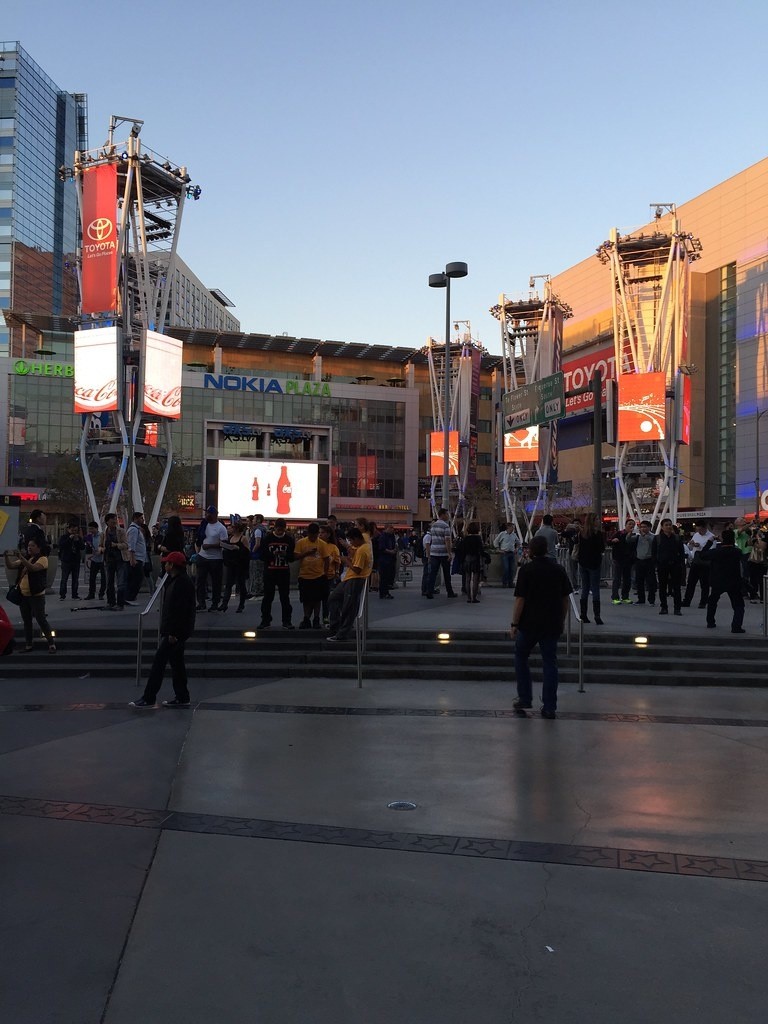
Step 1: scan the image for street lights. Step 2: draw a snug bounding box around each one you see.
[429,263,468,586]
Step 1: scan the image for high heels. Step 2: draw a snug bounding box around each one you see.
[580,616,604,625]
[216,604,245,613]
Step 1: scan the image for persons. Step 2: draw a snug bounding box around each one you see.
[420,508,767,633]
[129,551,196,709]
[509,535,571,720]
[0,506,414,652]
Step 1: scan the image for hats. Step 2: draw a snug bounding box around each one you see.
[734,517,746,525]
[202,506,218,514]
[160,552,186,566]
[153,523,160,530]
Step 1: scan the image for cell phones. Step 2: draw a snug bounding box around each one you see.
[275,551,282,553]
[312,548,317,552]
[6,550,17,555]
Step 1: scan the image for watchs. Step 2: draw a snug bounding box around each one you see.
[509,622,518,628]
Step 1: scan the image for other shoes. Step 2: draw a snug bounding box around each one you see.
[707,624,716,628]
[84,595,104,600]
[256,622,272,630]
[380,594,394,599]
[659,610,682,616]
[447,593,458,598]
[299,620,322,629]
[633,600,656,607]
[467,600,480,603]
[731,628,746,633]
[282,623,296,630]
[48,647,56,654]
[18,647,32,653]
[105,604,126,610]
[59,596,81,601]
[196,604,218,613]
[126,600,141,606]
[248,596,265,601]
[425,591,434,599]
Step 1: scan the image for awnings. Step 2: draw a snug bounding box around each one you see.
[105,515,413,533]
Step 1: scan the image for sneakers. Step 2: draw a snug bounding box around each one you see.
[327,636,348,641]
[611,598,633,605]
[513,697,533,708]
[540,705,556,717]
[129,696,191,709]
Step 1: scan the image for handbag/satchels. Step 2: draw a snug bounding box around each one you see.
[571,531,580,561]
[6,585,22,605]
[749,547,763,563]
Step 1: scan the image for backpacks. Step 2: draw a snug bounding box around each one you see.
[371,533,388,566]
[414,533,432,558]
[253,528,265,561]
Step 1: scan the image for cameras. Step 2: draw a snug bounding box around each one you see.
[714,537,718,540]
